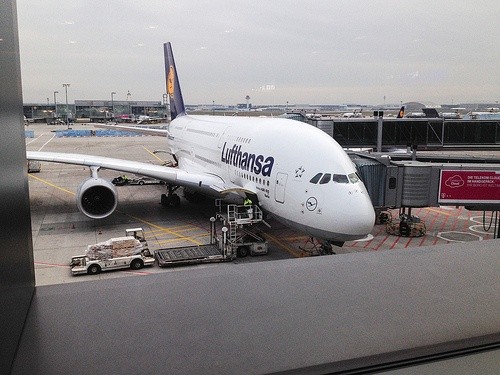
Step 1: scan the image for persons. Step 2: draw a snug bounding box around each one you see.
[242,196,255,220]
[250,193,259,220]
[398,216,412,238]
[121,175,130,185]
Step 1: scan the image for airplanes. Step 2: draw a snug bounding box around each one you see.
[25,42,500,254]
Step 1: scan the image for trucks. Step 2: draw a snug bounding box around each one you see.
[71,254,155,275]
